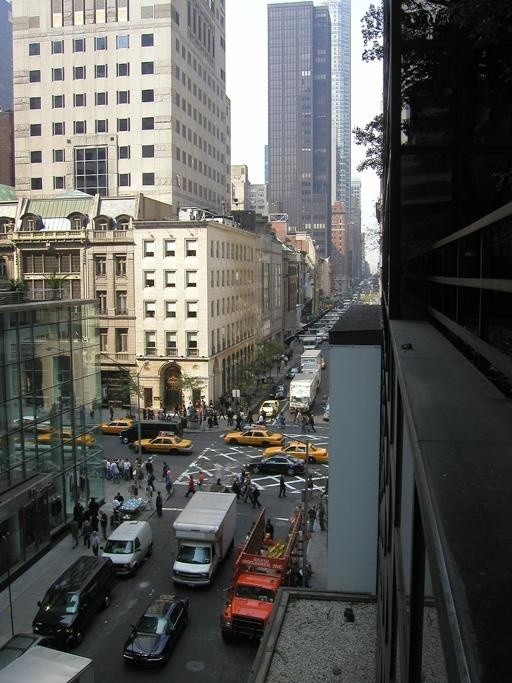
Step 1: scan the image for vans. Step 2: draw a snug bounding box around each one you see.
[33,555,117,646]
[1,634,52,672]
[99,520,154,577]
[122,594,192,665]
[303,336,317,350]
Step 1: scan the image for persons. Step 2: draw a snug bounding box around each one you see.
[295,408,317,433]
[308,502,328,532]
[265,519,274,540]
[277,472,286,497]
[223,403,286,430]
[70,455,173,556]
[144,398,219,427]
[184,469,262,510]
[109,404,114,421]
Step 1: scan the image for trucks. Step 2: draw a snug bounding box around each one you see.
[0,644,95,683]
[289,373,319,412]
[216,506,304,642]
[119,420,183,443]
[300,349,321,369]
[301,363,322,388]
[172,490,238,587]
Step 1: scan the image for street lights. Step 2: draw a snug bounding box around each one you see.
[261,427,316,500]
[46,347,65,403]
[136,360,150,479]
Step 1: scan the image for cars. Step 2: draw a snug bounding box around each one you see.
[242,455,305,475]
[274,383,286,399]
[286,366,300,378]
[309,277,373,343]
[100,417,135,434]
[259,400,279,417]
[128,431,194,455]
[13,415,51,431]
[225,424,284,446]
[1,434,35,448]
[321,355,326,368]
[36,426,96,448]
[262,440,328,463]
